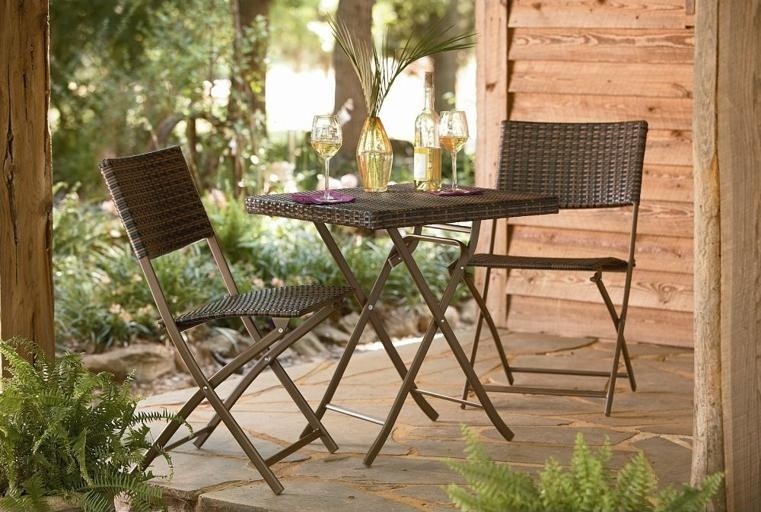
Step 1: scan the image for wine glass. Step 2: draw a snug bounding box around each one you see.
[439,110,470,194]
[310,114,341,202]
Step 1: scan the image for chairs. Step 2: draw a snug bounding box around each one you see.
[448,119,648,417]
[99,143,356,495]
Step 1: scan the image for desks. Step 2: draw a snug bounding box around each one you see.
[242,181,560,466]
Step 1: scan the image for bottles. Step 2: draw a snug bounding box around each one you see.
[413,70,443,194]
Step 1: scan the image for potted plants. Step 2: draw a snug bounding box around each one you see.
[324,8,481,194]
